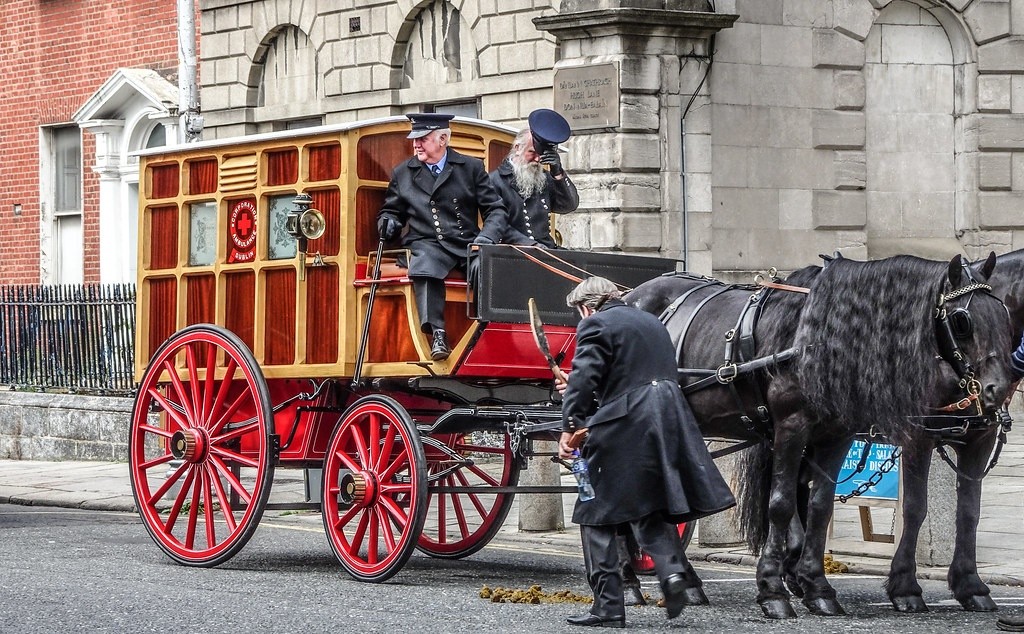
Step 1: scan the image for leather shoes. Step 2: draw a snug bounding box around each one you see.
[662,574,686,619]
[430,331,449,360]
[567,612,625,628]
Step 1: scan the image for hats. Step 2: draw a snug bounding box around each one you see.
[406,113,455,139]
[528,109,570,155]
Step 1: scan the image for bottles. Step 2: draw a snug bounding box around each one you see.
[572,450,595,502]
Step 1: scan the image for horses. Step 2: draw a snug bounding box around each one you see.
[620,246,1024,619]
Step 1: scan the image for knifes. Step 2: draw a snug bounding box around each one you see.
[529,297,567,389]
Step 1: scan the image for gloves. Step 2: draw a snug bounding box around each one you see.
[540,150,562,176]
[381,219,402,239]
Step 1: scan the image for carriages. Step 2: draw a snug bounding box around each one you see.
[128,113,1024,623]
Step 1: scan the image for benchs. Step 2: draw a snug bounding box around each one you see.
[355,245,473,365]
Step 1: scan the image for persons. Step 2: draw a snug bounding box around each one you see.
[558,276,736,627]
[375,108,579,361]
